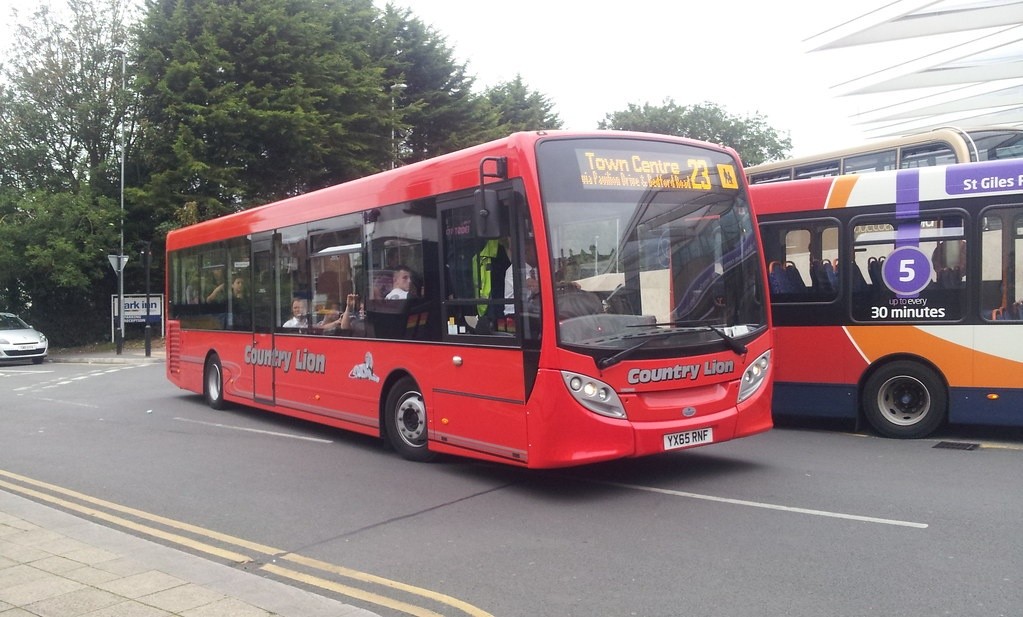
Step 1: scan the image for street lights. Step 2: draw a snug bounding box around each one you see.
[390,83,410,170]
[112,45,125,349]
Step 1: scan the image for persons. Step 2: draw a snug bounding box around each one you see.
[503,236,582,322]
[184,218,515,331]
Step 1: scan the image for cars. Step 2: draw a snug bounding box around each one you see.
[0,312,48,367]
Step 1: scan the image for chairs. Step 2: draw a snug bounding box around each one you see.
[768,256,1023,321]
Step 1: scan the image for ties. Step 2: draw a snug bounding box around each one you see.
[526,269,537,301]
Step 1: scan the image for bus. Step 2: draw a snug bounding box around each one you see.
[737,127,1021,257]
[670,156,1022,439]
[165,129,776,472]
